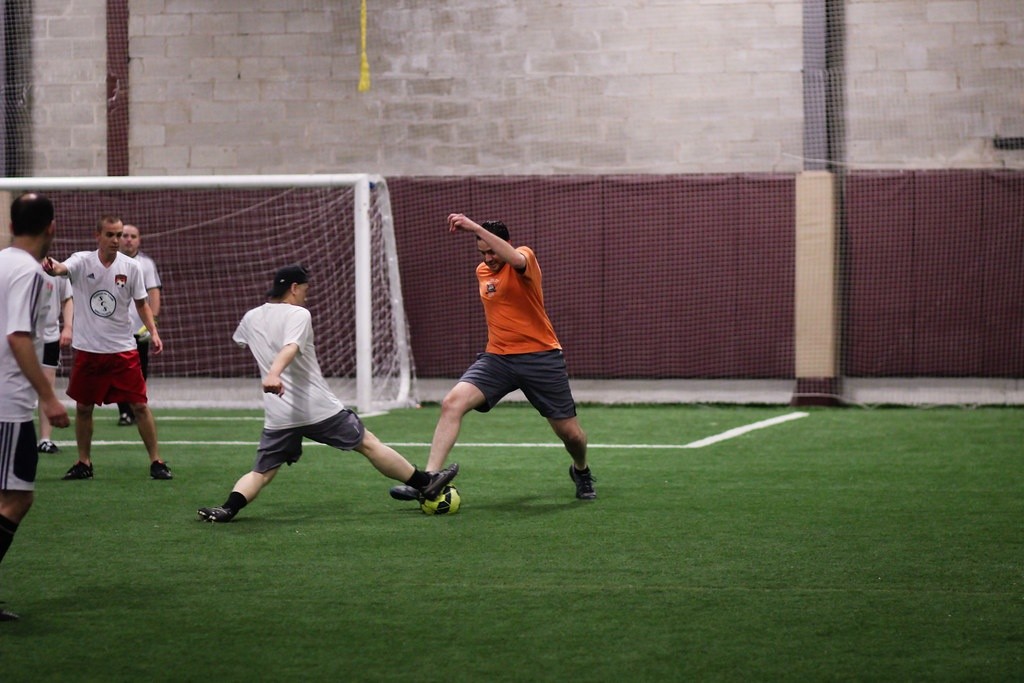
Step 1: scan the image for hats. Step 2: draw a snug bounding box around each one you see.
[268,265,308,297]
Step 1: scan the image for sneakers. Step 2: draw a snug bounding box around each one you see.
[390,485,425,503]
[117,412,134,425]
[0,607,21,624]
[149,459,173,479]
[418,462,459,501]
[62,460,93,480]
[569,463,598,500]
[38,441,58,453]
[197,507,234,523]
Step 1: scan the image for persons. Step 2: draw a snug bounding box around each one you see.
[195,266,460,523]
[41,214,173,480]
[389,213,599,502]
[38,256,75,455]
[117,224,163,427]
[0,193,71,620]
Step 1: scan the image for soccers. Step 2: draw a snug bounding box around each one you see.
[420,482,461,516]
